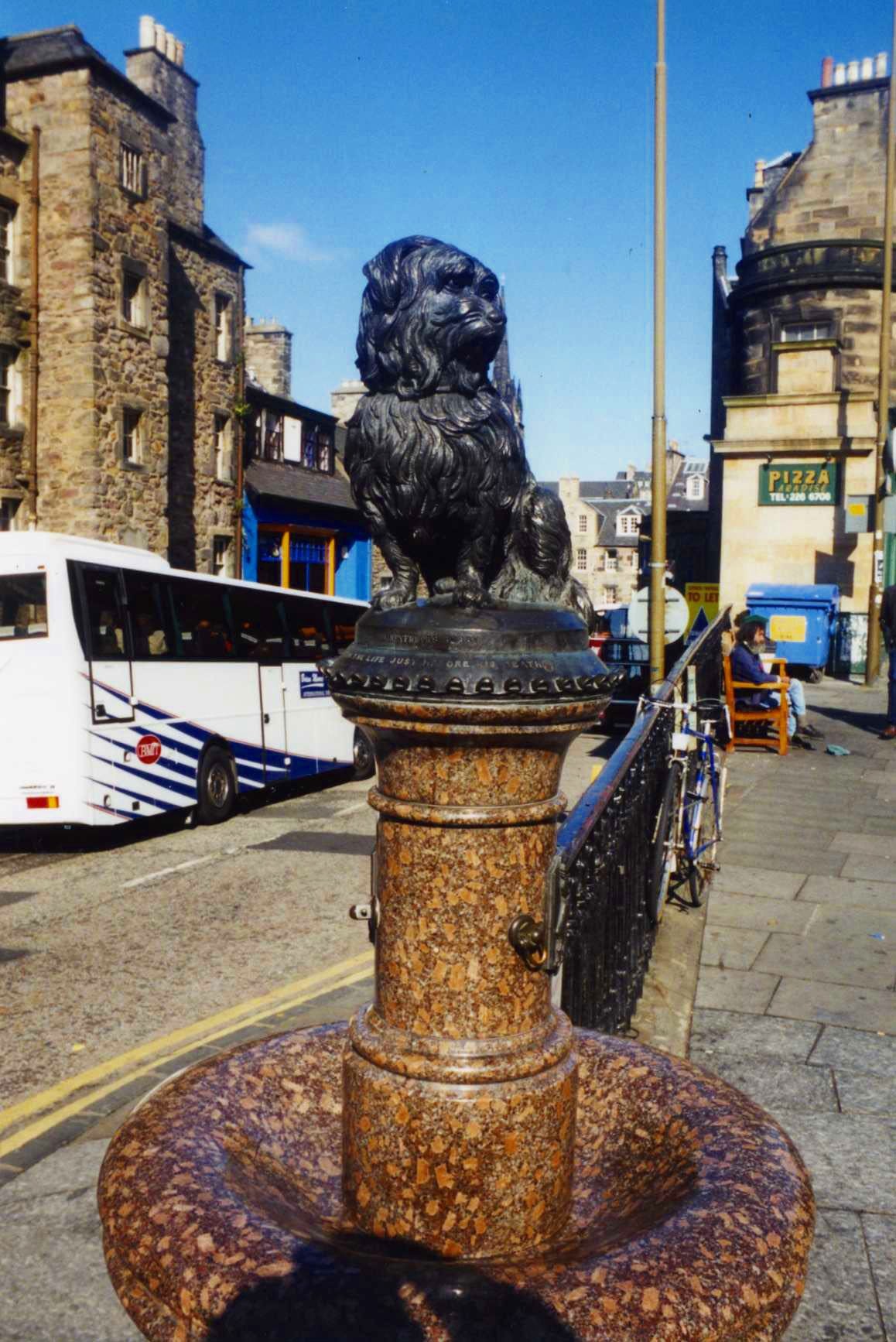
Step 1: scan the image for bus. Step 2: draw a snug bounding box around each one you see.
[0,528,377,827]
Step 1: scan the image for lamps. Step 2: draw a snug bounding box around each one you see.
[270,539,280,557]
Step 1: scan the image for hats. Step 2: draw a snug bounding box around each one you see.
[740,615,769,627]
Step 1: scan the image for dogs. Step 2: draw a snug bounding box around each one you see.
[345,235,595,632]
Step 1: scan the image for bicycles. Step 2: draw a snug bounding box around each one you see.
[634,678,733,924]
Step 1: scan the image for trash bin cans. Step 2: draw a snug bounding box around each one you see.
[744,582,839,682]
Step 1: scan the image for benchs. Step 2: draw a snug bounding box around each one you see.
[723,654,790,756]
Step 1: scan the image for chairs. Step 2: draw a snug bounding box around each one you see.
[0,623,352,662]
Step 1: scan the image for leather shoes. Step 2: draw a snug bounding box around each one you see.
[795,725,825,740]
[879,723,896,739]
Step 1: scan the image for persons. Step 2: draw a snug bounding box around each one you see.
[137,613,167,655]
[725,619,826,751]
[875,582,895,743]
[99,608,125,656]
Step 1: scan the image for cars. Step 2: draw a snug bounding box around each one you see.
[587,605,683,727]
